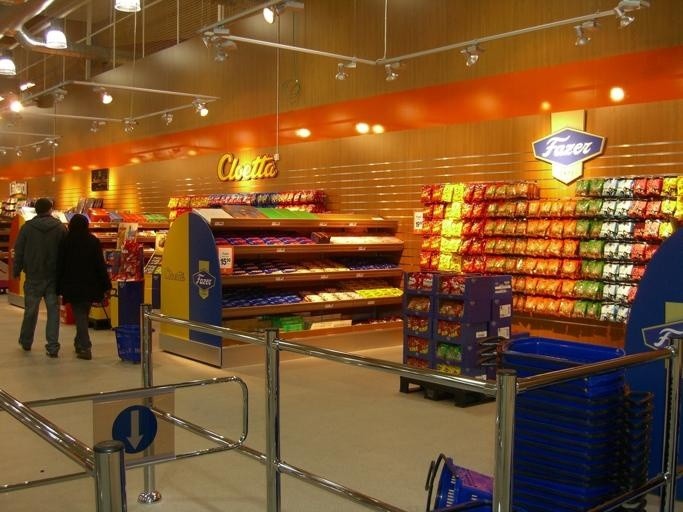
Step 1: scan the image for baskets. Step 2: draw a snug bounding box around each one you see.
[426,454,494,512]
[112,325,155,363]
[477,336,655,511]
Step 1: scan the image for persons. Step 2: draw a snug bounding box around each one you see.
[56,213,111,360]
[11,197,67,358]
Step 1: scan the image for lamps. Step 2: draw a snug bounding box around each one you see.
[459,46,481,68]
[207,37,230,63]
[43,18,69,52]
[613,2,647,29]
[262,6,284,24]
[192,102,212,118]
[572,18,599,46]
[113,0,143,15]
[101,93,114,105]
[384,63,400,83]
[88,119,108,133]
[162,112,175,125]
[11,100,24,115]
[2,56,19,79]
[122,120,139,133]
[1,137,60,157]
[334,59,357,80]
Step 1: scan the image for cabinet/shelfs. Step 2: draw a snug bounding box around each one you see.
[3,195,171,330]
[389,174,683,405]
[394,268,515,403]
[158,196,411,369]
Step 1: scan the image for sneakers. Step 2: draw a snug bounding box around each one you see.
[76,347,91,360]
[46,349,57,358]
[19,338,31,351]
